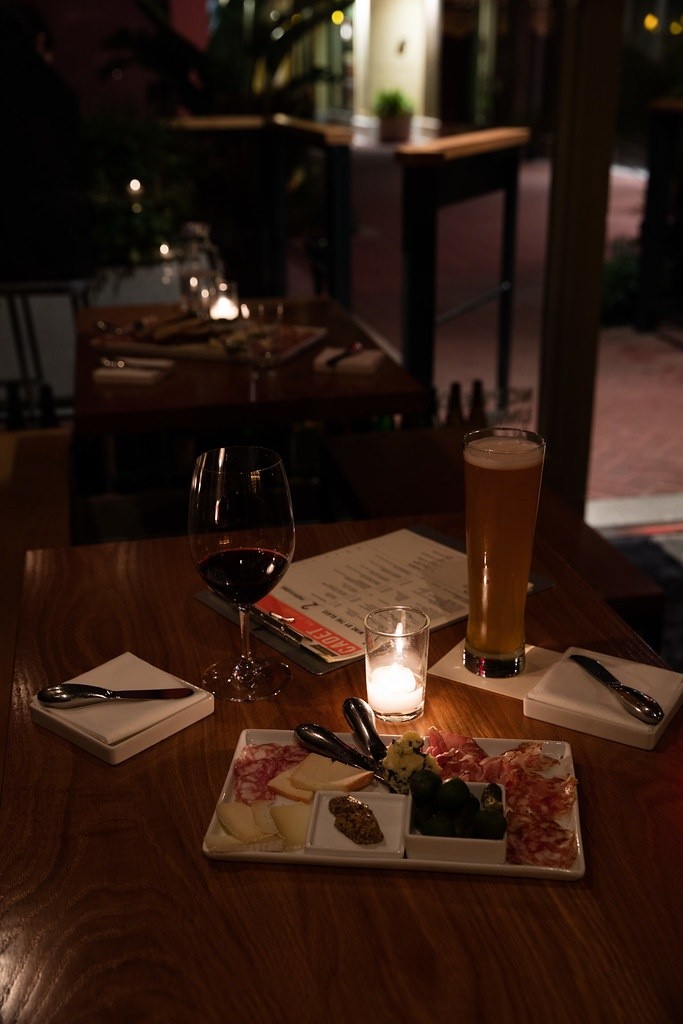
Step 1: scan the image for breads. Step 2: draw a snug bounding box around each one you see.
[266,751,375,804]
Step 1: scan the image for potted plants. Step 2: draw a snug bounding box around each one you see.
[374,93,412,139]
[132,0,357,246]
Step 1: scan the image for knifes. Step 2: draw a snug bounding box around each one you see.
[569,654,664,725]
[326,342,362,368]
[342,697,409,796]
[38,683,194,709]
[295,723,386,783]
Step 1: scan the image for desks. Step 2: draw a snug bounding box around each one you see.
[266,109,532,414]
[70,299,426,543]
[0,509,683,1024]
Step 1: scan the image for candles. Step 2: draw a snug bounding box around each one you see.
[361,603,431,724]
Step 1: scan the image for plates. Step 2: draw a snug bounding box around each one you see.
[305,790,408,858]
[90,315,328,363]
[203,729,585,881]
[406,781,506,863]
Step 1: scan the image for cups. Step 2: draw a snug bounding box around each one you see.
[365,606,430,724]
[463,427,546,678]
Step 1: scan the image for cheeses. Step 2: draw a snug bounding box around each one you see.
[203,800,312,853]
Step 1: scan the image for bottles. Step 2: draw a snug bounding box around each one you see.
[377,415,396,433]
[430,379,487,429]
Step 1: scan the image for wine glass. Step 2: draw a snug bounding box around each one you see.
[88,268,125,368]
[188,445,295,703]
[238,300,283,381]
